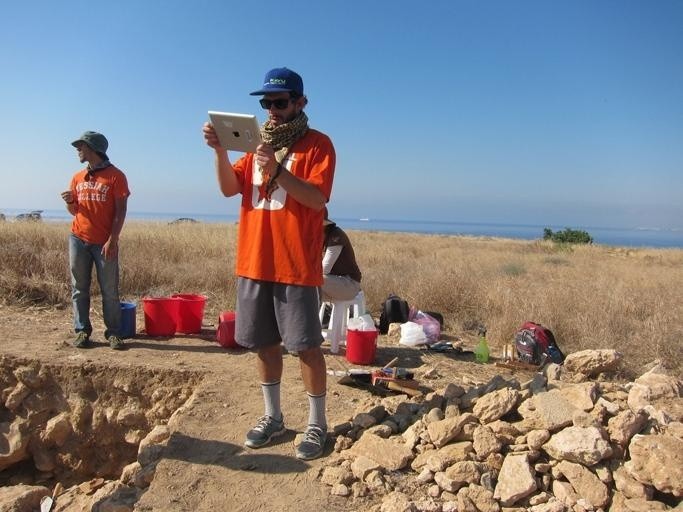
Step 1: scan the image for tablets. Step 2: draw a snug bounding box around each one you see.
[208,111,263,154]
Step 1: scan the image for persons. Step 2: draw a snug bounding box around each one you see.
[202,67,336,461]
[316,207,362,310]
[60,131,130,351]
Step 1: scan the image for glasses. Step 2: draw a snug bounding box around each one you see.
[259,96,294,110]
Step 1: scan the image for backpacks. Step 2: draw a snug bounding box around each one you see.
[516,321,565,365]
[380,294,409,334]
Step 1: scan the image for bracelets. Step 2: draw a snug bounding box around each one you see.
[67,200,74,204]
[269,162,282,183]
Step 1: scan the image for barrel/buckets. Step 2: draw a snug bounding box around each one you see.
[141,295,176,336]
[216,312,241,347]
[170,293,208,333]
[345,328,381,366]
[119,301,138,338]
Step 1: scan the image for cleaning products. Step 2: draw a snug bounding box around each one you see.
[475,323,489,364]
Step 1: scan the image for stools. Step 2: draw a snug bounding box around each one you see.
[319,289,365,355]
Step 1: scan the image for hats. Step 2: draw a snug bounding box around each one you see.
[71,131,109,160]
[249,66,303,95]
[323,206,336,227]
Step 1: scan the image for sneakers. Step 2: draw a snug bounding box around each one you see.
[244,412,286,449]
[108,334,123,349]
[296,423,328,461]
[72,331,89,347]
